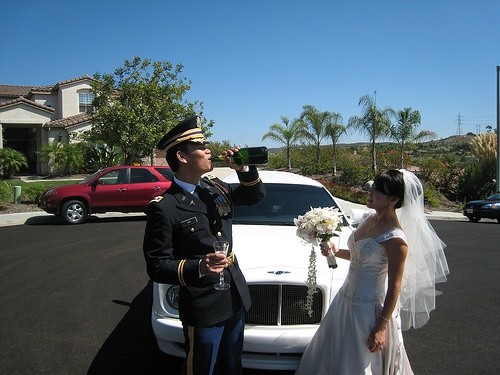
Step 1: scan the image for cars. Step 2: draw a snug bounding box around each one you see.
[462,190,500,223]
[150,167,368,371]
[36,164,173,224]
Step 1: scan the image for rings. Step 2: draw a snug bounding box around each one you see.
[379,346,384,349]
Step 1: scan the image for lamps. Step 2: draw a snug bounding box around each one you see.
[58,132,62,141]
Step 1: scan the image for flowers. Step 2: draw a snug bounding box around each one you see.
[294,205,344,269]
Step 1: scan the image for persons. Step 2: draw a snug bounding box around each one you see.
[294,168,450,375]
[142,115,266,375]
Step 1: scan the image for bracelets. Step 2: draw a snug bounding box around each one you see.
[379,315,389,322]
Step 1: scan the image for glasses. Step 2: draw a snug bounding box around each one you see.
[185,143,208,154]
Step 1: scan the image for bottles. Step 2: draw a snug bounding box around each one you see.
[211,147,268,166]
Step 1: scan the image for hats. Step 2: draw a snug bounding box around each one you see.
[157,115,209,154]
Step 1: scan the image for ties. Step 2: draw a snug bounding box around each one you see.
[194,186,210,206]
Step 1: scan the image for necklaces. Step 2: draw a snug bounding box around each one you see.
[368,220,376,232]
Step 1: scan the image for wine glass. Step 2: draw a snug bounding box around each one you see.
[213,240,231,290]
